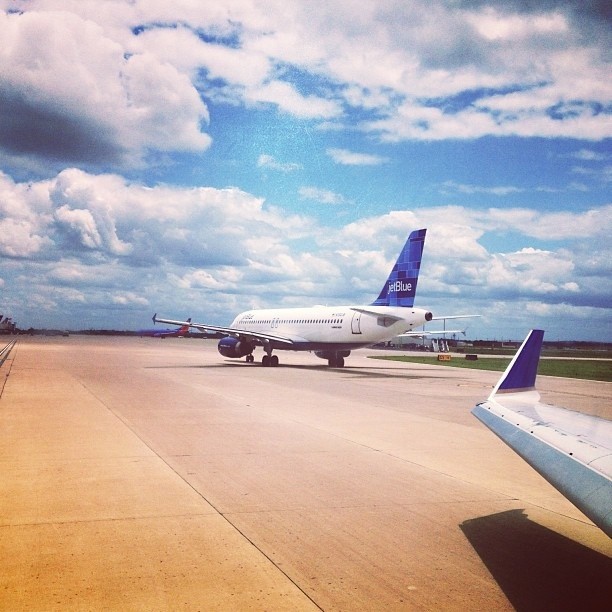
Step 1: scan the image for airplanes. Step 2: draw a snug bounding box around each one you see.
[152,229,465,368]
[136,318,191,339]
[470,329,611,539]
